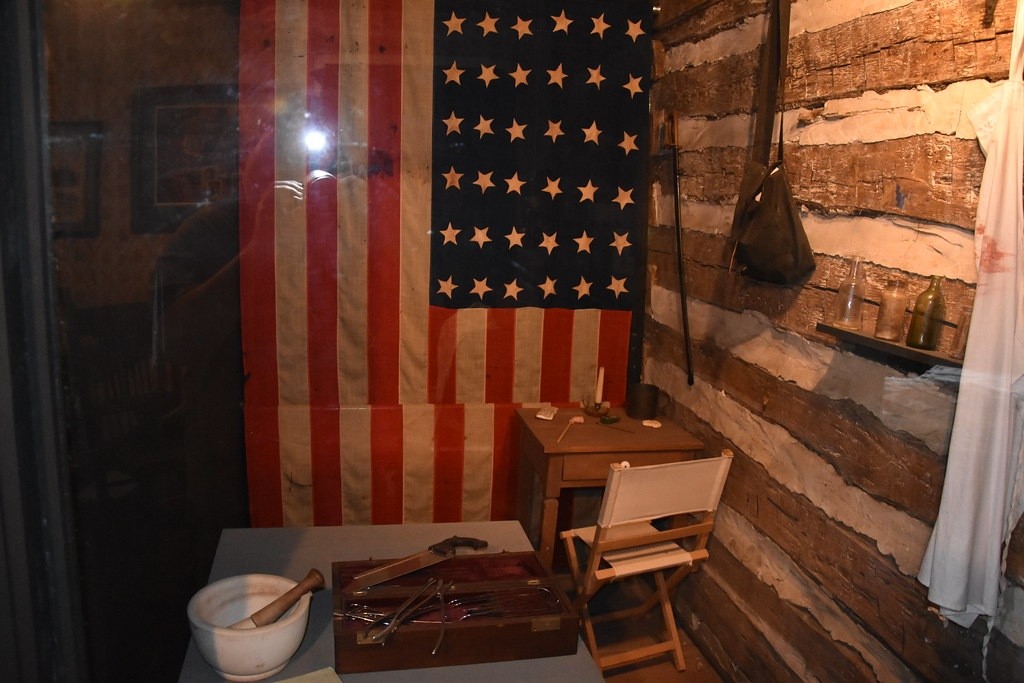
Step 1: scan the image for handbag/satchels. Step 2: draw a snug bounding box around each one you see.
[728,160,816,284]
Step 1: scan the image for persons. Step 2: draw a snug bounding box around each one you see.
[146,186,246,534]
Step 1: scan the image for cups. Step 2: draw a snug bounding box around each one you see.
[627,383,669,421]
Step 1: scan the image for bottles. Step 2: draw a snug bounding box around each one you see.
[906,275,944,351]
[948,306,973,358]
[873,280,907,343]
[831,256,865,332]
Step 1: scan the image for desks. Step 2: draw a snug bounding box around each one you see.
[178,520,604,683]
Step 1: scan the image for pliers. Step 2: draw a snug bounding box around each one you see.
[363,577,457,655]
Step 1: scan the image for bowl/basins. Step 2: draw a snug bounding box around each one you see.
[187,574,311,681]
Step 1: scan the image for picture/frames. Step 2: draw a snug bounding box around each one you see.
[128,84,240,233]
[47,119,107,239]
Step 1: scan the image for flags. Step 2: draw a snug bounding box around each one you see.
[240,0,652,526]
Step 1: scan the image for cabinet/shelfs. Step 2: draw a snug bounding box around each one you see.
[519,401,704,629]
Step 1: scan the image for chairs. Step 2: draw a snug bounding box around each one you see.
[561,448,735,676]
